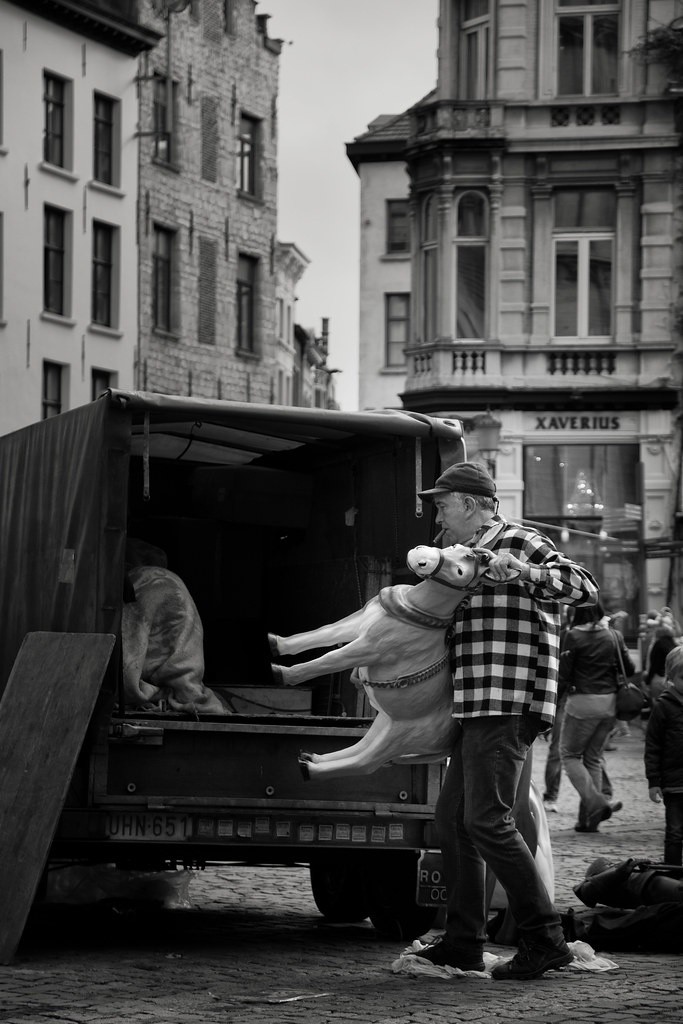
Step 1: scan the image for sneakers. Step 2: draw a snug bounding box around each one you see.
[490,938,575,981]
[403,934,487,973]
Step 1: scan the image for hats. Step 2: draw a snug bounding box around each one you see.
[416,461,497,504]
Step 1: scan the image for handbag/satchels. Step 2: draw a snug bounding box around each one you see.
[614,682,646,722]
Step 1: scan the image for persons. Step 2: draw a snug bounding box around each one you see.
[410,463,600,982]
[644,645,683,866]
[544,587,635,835]
[643,606,683,705]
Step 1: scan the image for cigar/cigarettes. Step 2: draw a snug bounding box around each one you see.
[433,529,445,544]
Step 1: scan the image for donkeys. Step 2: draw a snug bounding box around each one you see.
[268,539,521,781]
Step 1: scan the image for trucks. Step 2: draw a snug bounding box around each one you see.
[0,386,473,942]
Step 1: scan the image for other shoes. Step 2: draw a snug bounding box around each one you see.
[542,798,560,814]
[572,819,597,833]
[604,741,618,752]
[617,724,632,737]
[607,797,624,813]
[585,806,613,831]
